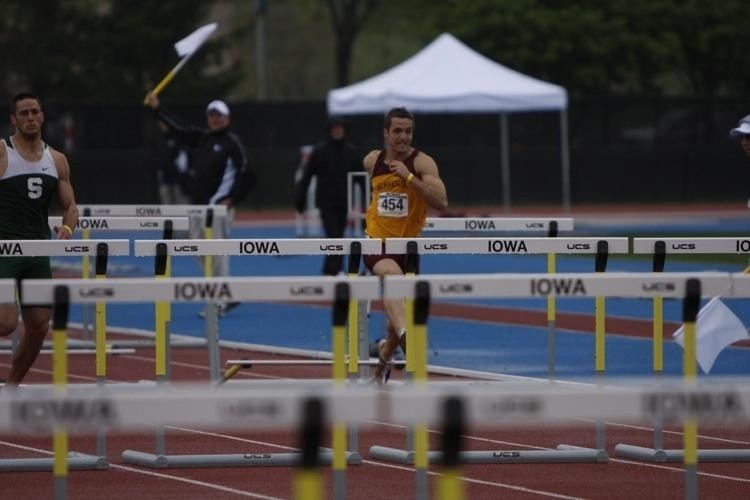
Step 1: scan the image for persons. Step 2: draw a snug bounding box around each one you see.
[150,117,187,208]
[143,89,248,319]
[725,110,750,295]
[0,87,83,392]
[360,103,453,386]
[287,117,366,280]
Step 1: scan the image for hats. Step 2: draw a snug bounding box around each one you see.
[205,99,230,116]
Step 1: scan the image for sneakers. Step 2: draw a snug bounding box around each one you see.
[197,296,240,319]
[400,328,408,354]
[369,338,394,383]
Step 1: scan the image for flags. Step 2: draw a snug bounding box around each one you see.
[171,19,219,65]
[667,297,749,380]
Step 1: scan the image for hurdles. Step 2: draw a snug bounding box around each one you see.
[388,380,750,499]
[1,279,111,472]
[1,379,384,500]
[133,239,384,378]
[46,215,213,350]
[1,239,156,388]
[69,202,228,324]
[613,271,749,464]
[418,215,589,387]
[384,236,628,388]
[21,275,384,470]
[633,234,750,376]
[367,273,613,466]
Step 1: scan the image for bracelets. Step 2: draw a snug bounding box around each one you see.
[404,171,416,187]
[60,223,73,236]
